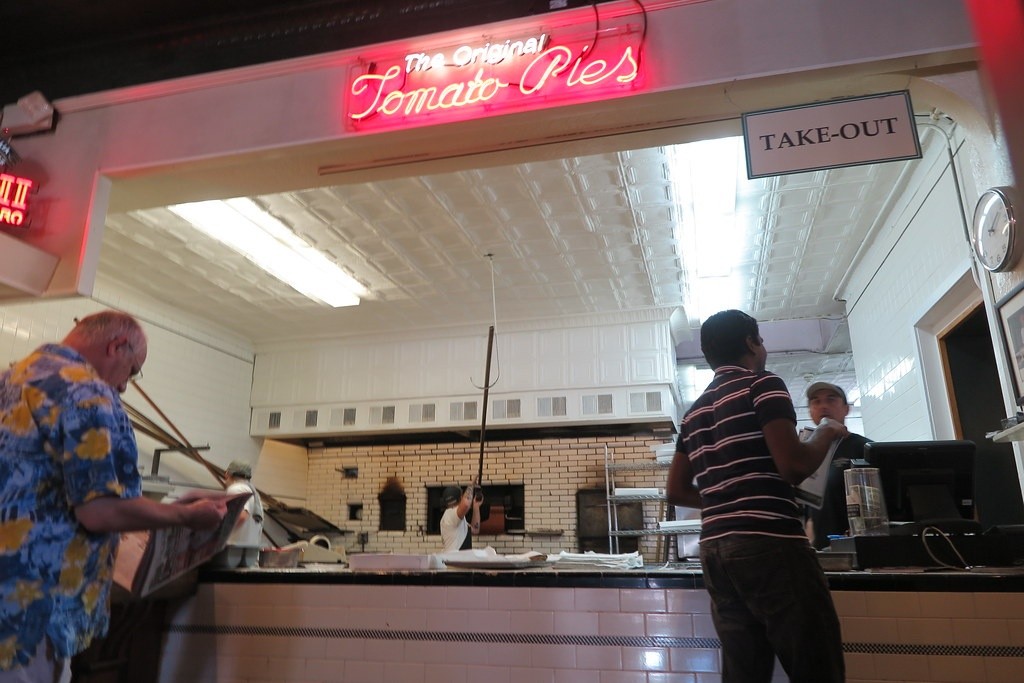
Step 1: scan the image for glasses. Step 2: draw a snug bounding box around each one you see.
[114,335,143,383]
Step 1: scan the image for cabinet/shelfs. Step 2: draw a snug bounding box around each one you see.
[604,444,701,555]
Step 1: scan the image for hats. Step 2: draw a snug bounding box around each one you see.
[443,486,462,505]
[807,382,847,405]
[226,459,252,479]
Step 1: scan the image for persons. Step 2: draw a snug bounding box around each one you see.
[440,477,484,553]
[665,311,850,683]
[802,381,873,549]
[222,460,265,570]
[0,310,226,683]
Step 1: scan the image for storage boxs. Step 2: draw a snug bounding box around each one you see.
[261,550,298,567]
[349,554,435,569]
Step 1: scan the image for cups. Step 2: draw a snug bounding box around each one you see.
[844,467,890,536]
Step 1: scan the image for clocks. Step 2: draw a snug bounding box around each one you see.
[971,185,1024,273]
